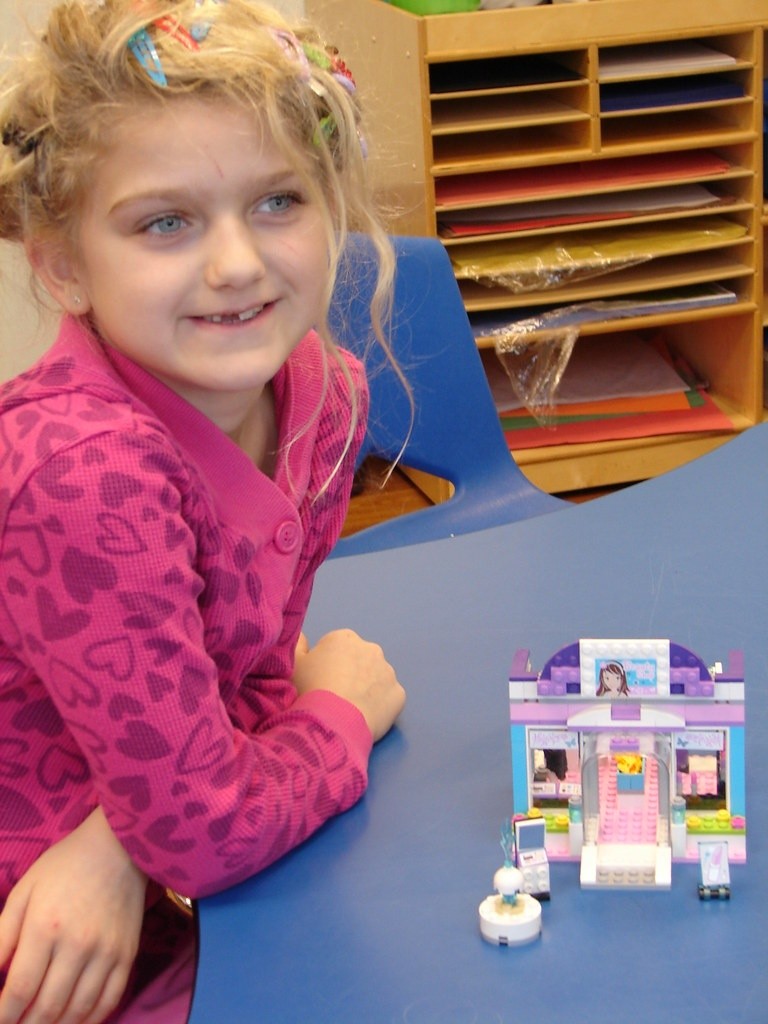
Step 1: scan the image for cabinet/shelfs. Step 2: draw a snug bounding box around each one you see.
[303,0,768,492]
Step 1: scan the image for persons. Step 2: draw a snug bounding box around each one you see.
[0,0,407,1023]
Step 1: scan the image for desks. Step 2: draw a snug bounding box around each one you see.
[190,418,768,1024]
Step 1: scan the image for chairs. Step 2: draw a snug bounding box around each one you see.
[313,232,579,561]
